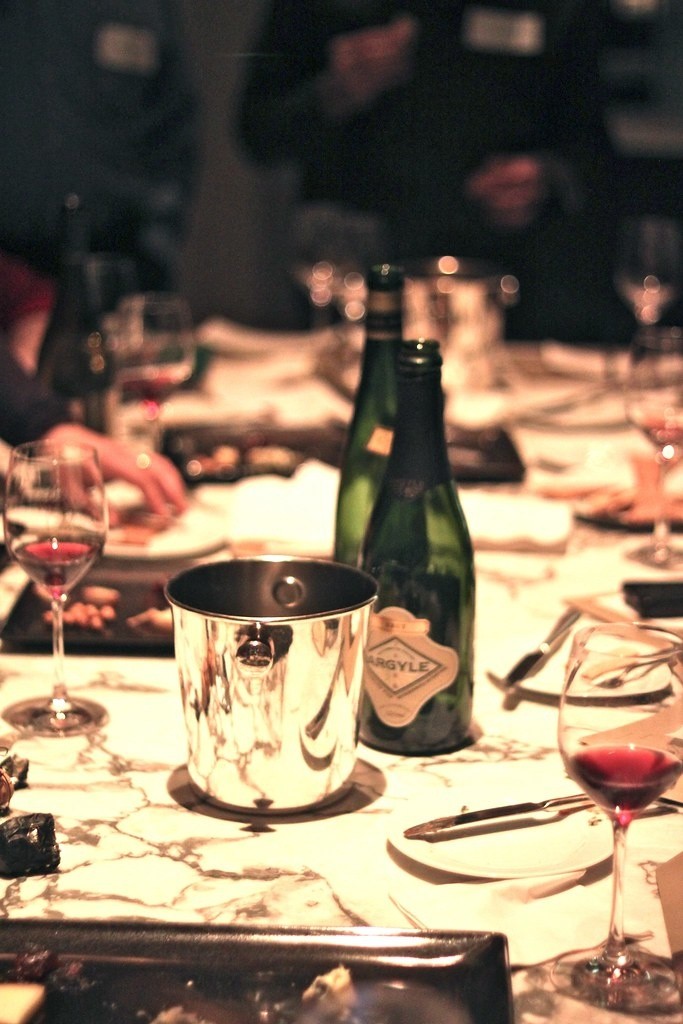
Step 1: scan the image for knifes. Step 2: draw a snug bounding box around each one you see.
[500,606,591,687]
[400,790,628,842]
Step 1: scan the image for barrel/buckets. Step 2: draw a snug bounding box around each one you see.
[165,556,379,816]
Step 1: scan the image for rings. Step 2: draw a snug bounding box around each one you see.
[138,454,151,468]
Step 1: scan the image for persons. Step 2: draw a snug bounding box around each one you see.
[0,1,682,528]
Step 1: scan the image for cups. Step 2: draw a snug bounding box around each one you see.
[120,291,197,395]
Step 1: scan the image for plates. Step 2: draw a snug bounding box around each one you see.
[478,615,671,698]
[2,910,518,1024]
[1,545,261,654]
[389,779,619,878]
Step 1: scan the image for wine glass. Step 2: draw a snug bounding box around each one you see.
[626,327,683,569]
[1,441,107,736]
[551,622,682,1008]
[616,224,682,362]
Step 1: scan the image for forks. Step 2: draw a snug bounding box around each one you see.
[583,643,682,690]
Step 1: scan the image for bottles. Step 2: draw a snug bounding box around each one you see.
[331,266,410,578]
[355,346,476,757]
